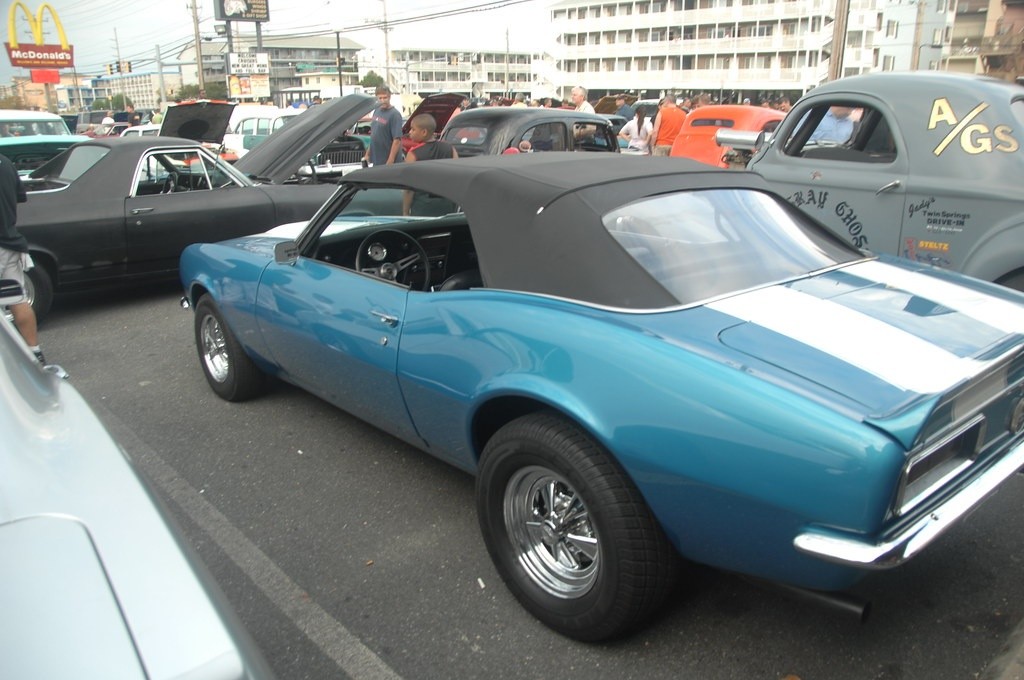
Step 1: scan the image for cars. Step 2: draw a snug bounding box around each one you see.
[0,94,380,334]
[593,115,629,148]
[0,135,95,182]
[631,99,683,118]
[58,99,310,165]
[716,71,1024,295]
[669,105,789,171]
[435,107,621,161]
[0,109,70,137]
[181,150,1023,645]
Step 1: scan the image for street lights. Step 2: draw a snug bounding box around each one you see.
[333,25,344,97]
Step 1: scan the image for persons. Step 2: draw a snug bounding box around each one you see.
[198,88,206,99]
[613,95,635,120]
[364,84,404,166]
[807,107,855,145]
[402,113,460,215]
[652,94,715,157]
[489,84,596,117]
[0,152,46,364]
[619,105,652,154]
[285,95,321,110]
[743,95,793,113]
[102,104,162,124]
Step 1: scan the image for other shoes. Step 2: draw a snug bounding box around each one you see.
[34,351,46,367]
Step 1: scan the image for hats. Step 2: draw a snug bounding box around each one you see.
[760,98,771,102]
[615,96,624,100]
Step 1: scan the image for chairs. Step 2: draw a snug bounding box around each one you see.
[438,268,482,290]
